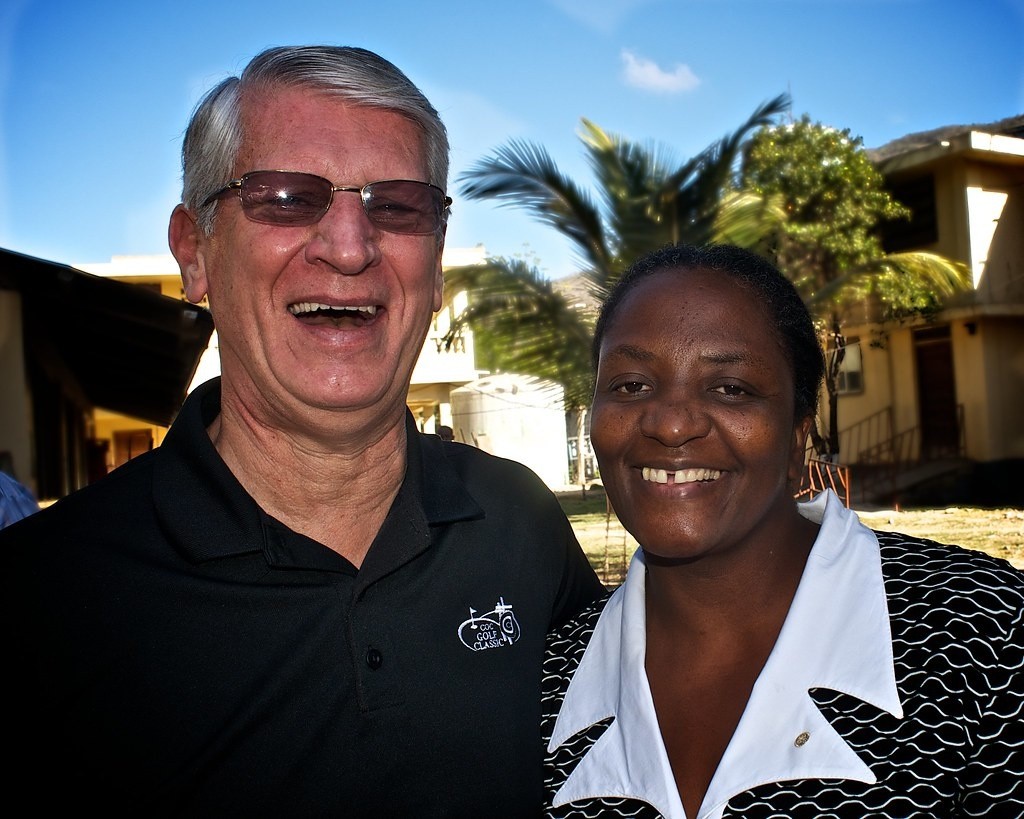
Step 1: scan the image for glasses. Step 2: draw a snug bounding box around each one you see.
[192,171,452,236]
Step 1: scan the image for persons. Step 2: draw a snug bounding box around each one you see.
[537,244,1024,819]
[0,45,602,819]
